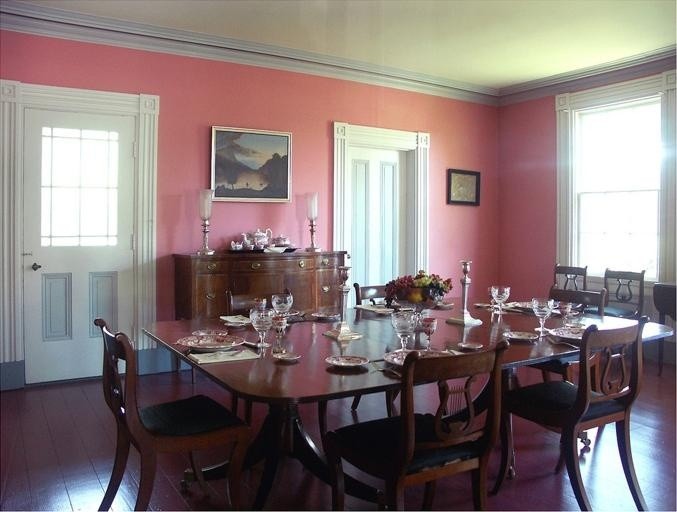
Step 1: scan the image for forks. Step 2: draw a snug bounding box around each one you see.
[205,348,243,356]
[370,360,401,377]
[545,334,579,350]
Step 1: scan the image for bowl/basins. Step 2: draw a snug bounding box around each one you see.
[267,247,287,253]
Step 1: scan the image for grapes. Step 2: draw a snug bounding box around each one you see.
[384,274,453,309]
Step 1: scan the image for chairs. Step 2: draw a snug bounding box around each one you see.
[549,262,587,311]
[584,265,649,376]
[531,284,606,395]
[222,289,291,423]
[91,314,247,512]
[322,340,510,512]
[492,312,675,512]
[348,284,400,415]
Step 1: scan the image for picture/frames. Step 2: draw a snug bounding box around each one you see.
[206,122,295,206]
[445,165,480,209]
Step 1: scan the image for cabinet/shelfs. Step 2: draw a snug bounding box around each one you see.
[170,250,346,324]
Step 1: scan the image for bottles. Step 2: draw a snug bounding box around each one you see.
[272,235,289,246]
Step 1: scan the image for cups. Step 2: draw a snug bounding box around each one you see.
[229,241,275,251]
[400,304,416,313]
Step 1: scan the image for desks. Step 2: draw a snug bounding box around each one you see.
[652,282,676,376]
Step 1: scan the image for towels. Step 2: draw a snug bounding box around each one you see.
[187,346,261,366]
[218,313,253,325]
[355,302,393,315]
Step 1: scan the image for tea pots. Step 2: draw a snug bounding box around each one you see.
[240,227,272,247]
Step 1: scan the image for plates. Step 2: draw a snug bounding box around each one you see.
[550,326,585,340]
[272,352,302,361]
[262,310,299,318]
[219,314,251,323]
[501,331,539,342]
[310,312,340,320]
[192,330,228,336]
[383,349,417,367]
[504,300,579,317]
[286,249,298,253]
[472,303,489,307]
[355,304,394,314]
[457,342,483,349]
[176,334,244,353]
[436,302,454,307]
[323,356,369,370]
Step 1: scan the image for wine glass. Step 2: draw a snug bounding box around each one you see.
[390,312,418,351]
[249,308,273,348]
[421,318,437,341]
[271,294,293,333]
[491,286,511,315]
[531,298,554,336]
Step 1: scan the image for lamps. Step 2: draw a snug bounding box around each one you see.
[197,189,215,256]
[303,188,321,253]
